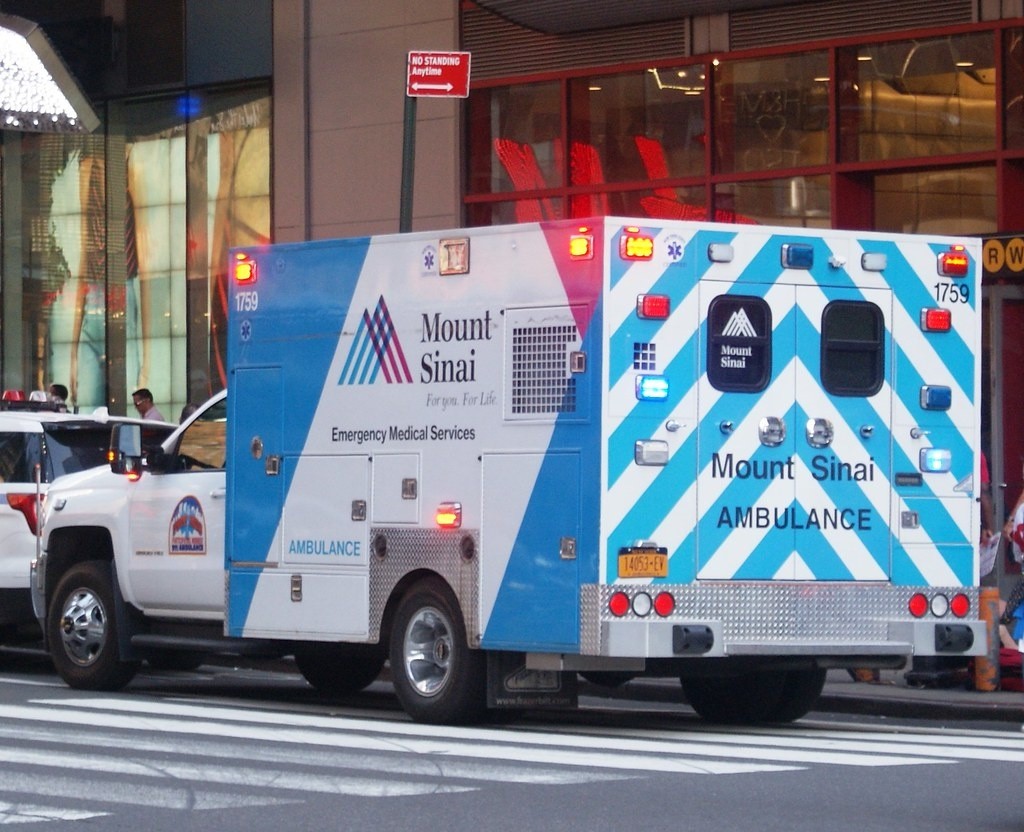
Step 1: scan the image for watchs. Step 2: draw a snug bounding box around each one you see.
[1008,516,1013,521]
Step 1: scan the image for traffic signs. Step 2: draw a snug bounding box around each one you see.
[405,50,470,97]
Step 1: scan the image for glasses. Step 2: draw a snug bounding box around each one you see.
[135,399,146,406]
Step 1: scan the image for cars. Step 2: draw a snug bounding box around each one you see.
[0,388,187,630]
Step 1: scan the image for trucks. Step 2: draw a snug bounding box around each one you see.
[27,215,992,730]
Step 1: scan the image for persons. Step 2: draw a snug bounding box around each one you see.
[40,384,72,413]
[131,389,164,422]
[177,403,200,425]
[980,449,1024,647]
